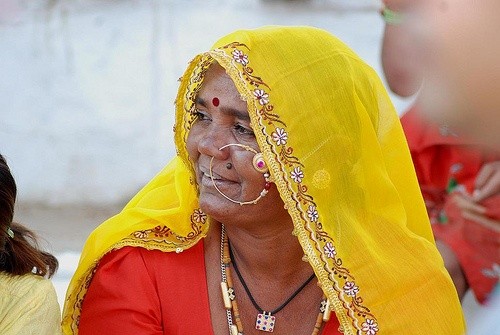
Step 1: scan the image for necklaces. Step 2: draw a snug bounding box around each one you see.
[221,222,332,335]
[227,233,316,333]
[223,230,327,335]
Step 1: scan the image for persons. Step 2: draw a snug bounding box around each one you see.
[61,27,466,335]
[379,1,500,307]
[0,154,61,333]
